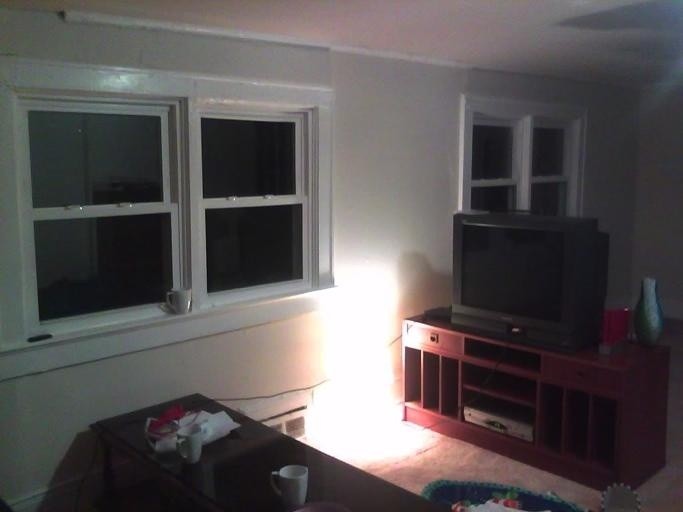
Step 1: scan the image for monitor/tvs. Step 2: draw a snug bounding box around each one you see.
[452,212,609,352]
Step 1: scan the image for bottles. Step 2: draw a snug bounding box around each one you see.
[636,275,663,348]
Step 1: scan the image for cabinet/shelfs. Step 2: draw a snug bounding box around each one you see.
[398,309,670,494]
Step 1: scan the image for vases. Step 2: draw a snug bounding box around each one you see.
[631,277,665,349]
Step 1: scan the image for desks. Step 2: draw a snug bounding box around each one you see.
[87,394,445,511]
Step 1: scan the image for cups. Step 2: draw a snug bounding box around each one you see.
[174,425,204,465]
[263,464,309,503]
[602,307,629,348]
[167,286,191,314]
